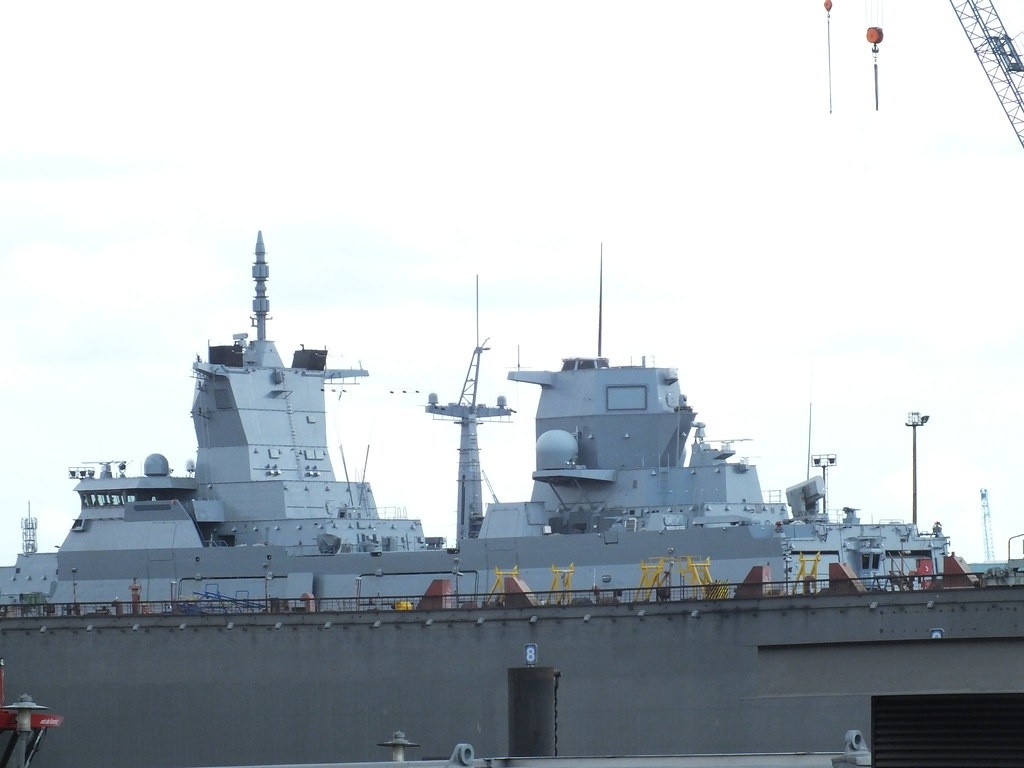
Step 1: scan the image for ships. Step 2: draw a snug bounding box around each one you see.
[0,227,1023,768]
